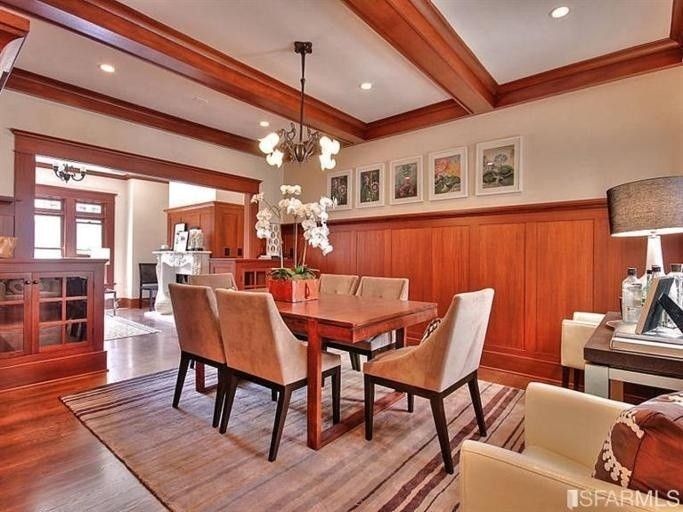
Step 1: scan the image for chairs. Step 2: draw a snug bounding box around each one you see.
[560,311,606,391]
[138,262,158,311]
[458,381,683,512]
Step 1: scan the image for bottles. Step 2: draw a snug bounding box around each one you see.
[645,265,683,327]
[621,268,642,322]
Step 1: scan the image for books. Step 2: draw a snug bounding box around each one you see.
[613,319,683,345]
[609,330,683,360]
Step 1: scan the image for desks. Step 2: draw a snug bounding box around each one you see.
[583,310,683,402]
[104,289,117,317]
[152,250,213,314]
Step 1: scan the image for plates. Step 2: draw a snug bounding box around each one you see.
[40,291,61,298]
[4,294,22,299]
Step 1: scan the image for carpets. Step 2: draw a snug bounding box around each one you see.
[104,309,163,341]
[58,340,526,512]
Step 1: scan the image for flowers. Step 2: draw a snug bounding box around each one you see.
[250,184,338,278]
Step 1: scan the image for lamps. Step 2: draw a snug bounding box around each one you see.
[257,41,341,171]
[606,175,683,283]
[89,247,117,290]
[53,158,88,184]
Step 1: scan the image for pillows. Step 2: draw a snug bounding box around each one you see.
[591,389,683,504]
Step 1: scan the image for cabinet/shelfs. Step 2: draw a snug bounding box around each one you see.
[0,258,109,391]
[163,200,294,291]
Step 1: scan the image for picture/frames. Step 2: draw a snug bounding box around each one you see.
[326,135,524,212]
[635,277,683,335]
